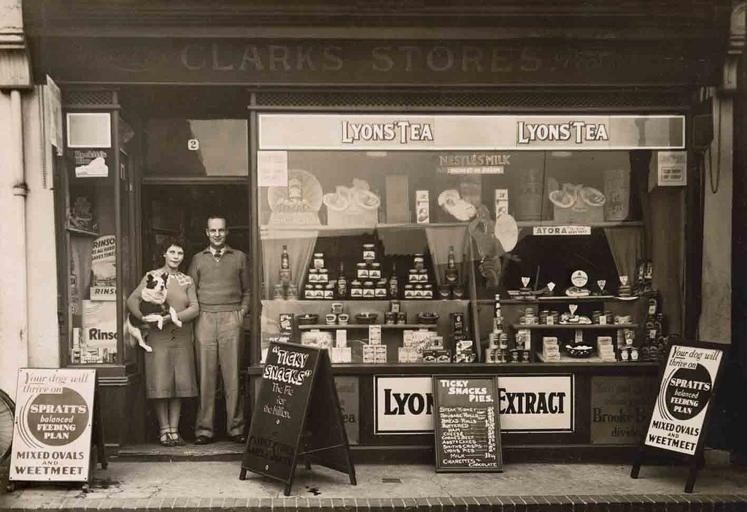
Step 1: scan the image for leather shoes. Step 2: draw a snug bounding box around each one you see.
[230,433,250,444]
[194,435,214,446]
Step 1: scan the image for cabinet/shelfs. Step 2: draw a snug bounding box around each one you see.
[245,86,695,447]
[53,85,142,445]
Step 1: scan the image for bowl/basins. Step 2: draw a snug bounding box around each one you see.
[415,312,441,324]
[355,312,378,325]
[294,312,321,324]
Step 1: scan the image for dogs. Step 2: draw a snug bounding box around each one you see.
[126,271,183,352]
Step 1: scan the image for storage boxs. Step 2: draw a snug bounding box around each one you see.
[577,374,663,446]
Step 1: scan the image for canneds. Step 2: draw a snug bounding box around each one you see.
[272,243,464,325]
[591,310,602,324]
[486,307,559,364]
[603,310,613,324]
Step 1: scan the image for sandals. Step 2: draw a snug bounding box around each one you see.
[159,428,188,447]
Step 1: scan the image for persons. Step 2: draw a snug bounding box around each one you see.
[188,212,251,445]
[126,237,201,447]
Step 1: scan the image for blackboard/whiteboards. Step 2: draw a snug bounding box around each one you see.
[241,341,323,485]
[433,374,503,473]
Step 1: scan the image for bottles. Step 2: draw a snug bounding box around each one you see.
[494,293,501,317]
[448,245,456,269]
[280,245,290,269]
